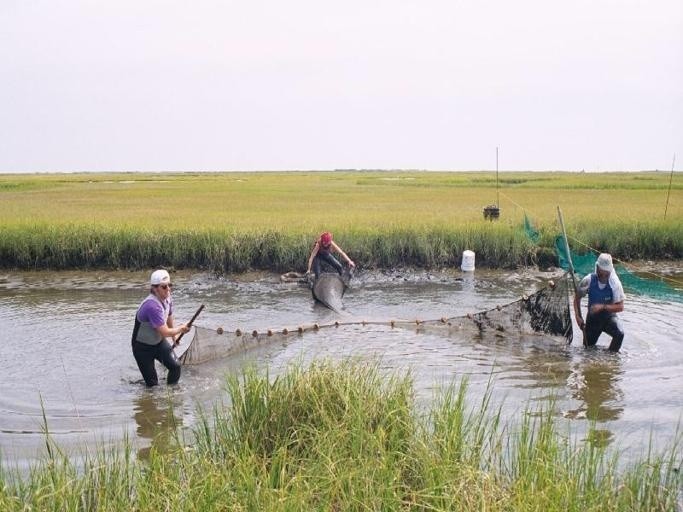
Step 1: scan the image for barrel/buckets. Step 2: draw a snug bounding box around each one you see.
[461,249,476,272]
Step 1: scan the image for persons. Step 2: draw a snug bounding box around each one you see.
[306,232,356,279]
[573,253,626,352]
[131,270,191,387]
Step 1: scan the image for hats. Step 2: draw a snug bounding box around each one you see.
[597,253,612,271]
[322,231,332,243]
[151,270,174,285]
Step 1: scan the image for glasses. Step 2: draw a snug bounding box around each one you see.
[160,283,173,288]
